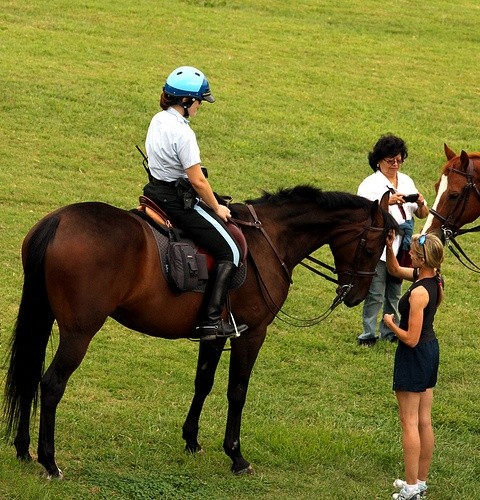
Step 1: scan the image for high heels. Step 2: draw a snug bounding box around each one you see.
[392,478,427,500]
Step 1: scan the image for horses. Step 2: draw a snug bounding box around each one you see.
[421,143,480,248]
[0,183,404,478]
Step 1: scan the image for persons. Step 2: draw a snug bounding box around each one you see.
[384,228,444,500]
[356,135,430,345]
[144,66,250,341]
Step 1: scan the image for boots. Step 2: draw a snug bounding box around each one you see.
[199,261,248,340]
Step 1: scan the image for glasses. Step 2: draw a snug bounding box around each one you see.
[384,159,405,165]
[417,235,430,262]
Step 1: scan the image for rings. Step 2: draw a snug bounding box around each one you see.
[397,200,400,203]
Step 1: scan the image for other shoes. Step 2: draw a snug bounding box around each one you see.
[360,336,379,346]
[382,336,398,343]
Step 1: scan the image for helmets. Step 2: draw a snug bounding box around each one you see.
[163,66,216,103]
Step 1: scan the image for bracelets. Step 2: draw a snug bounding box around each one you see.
[418,200,427,207]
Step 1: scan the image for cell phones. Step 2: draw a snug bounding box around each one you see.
[403,194,420,202]
